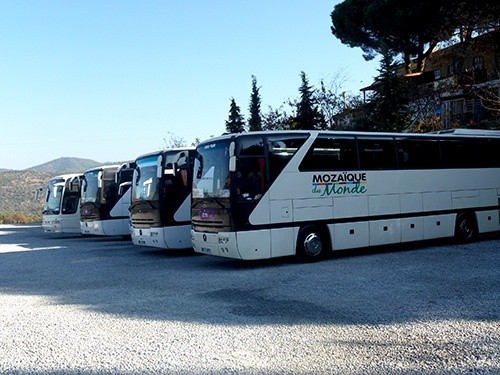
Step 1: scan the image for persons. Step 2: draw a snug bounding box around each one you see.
[222,176,240,194]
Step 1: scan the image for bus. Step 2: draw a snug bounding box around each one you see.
[173,129,500,263]
[69,163,132,240]
[115,148,196,249]
[35,174,89,236]
